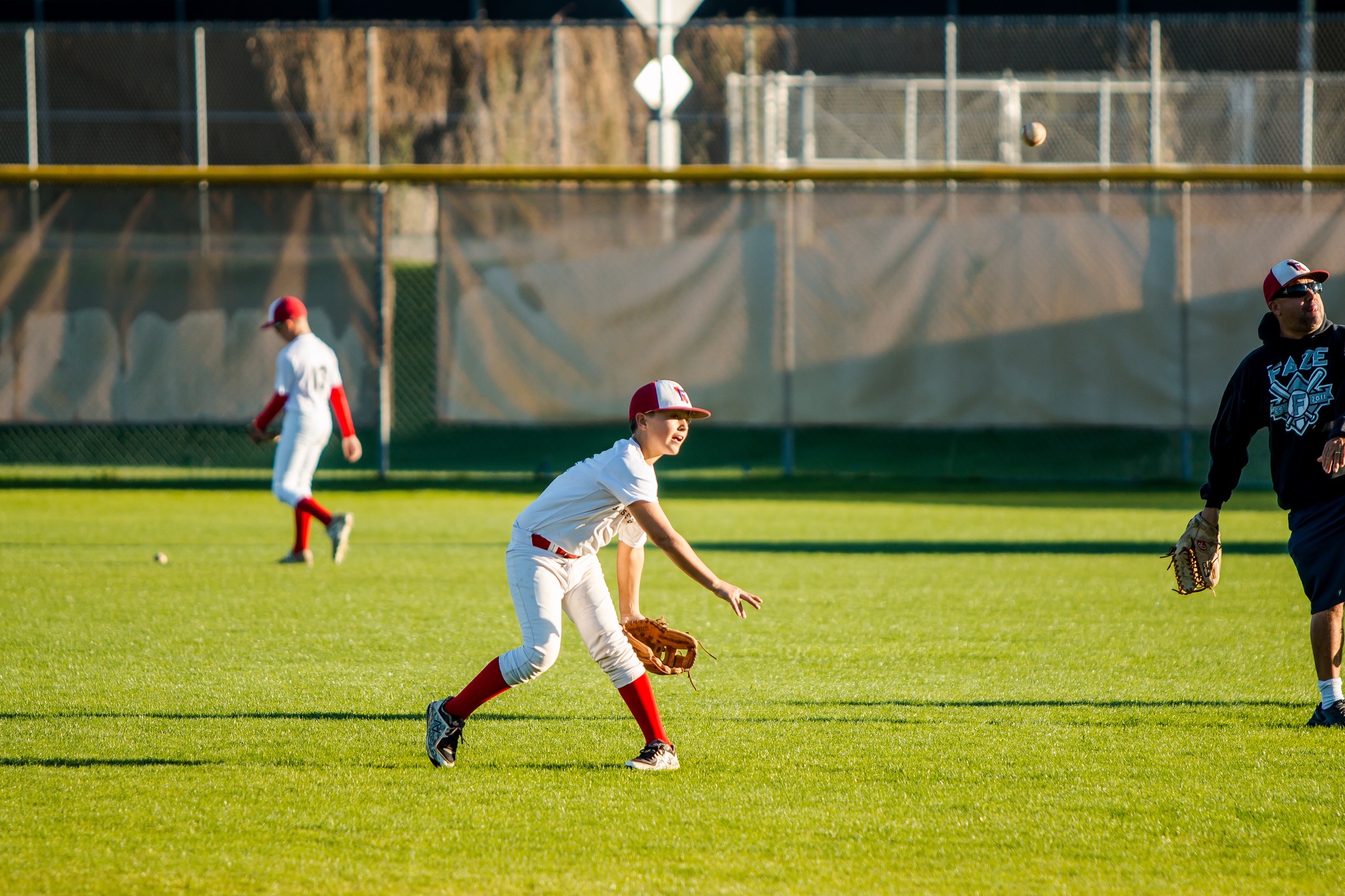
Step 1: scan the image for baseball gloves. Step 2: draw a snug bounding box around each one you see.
[1171,510,1222,595]
[621,617,698,676]
[248,423,279,443]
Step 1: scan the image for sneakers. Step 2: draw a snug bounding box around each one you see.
[425,696,469,768]
[276,549,312,563]
[327,515,353,564]
[1306,699,1345,727]
[625,738,682,770]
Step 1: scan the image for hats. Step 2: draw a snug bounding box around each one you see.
[260,296,308,329]
[1263,259,1330,305]
[630,379,711,419]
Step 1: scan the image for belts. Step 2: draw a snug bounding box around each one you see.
[532,533,583,559]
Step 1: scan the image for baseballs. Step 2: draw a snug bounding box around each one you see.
[1020,121,1047,147]
[153,552,168,564]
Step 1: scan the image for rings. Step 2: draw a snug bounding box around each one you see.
[1335,452,1340,453]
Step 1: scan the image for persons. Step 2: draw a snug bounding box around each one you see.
[426,380,763,771]
[1171,259,1345,728]
[246,296,363,563]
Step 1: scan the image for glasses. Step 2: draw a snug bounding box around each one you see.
[1273,281,1324,298]
